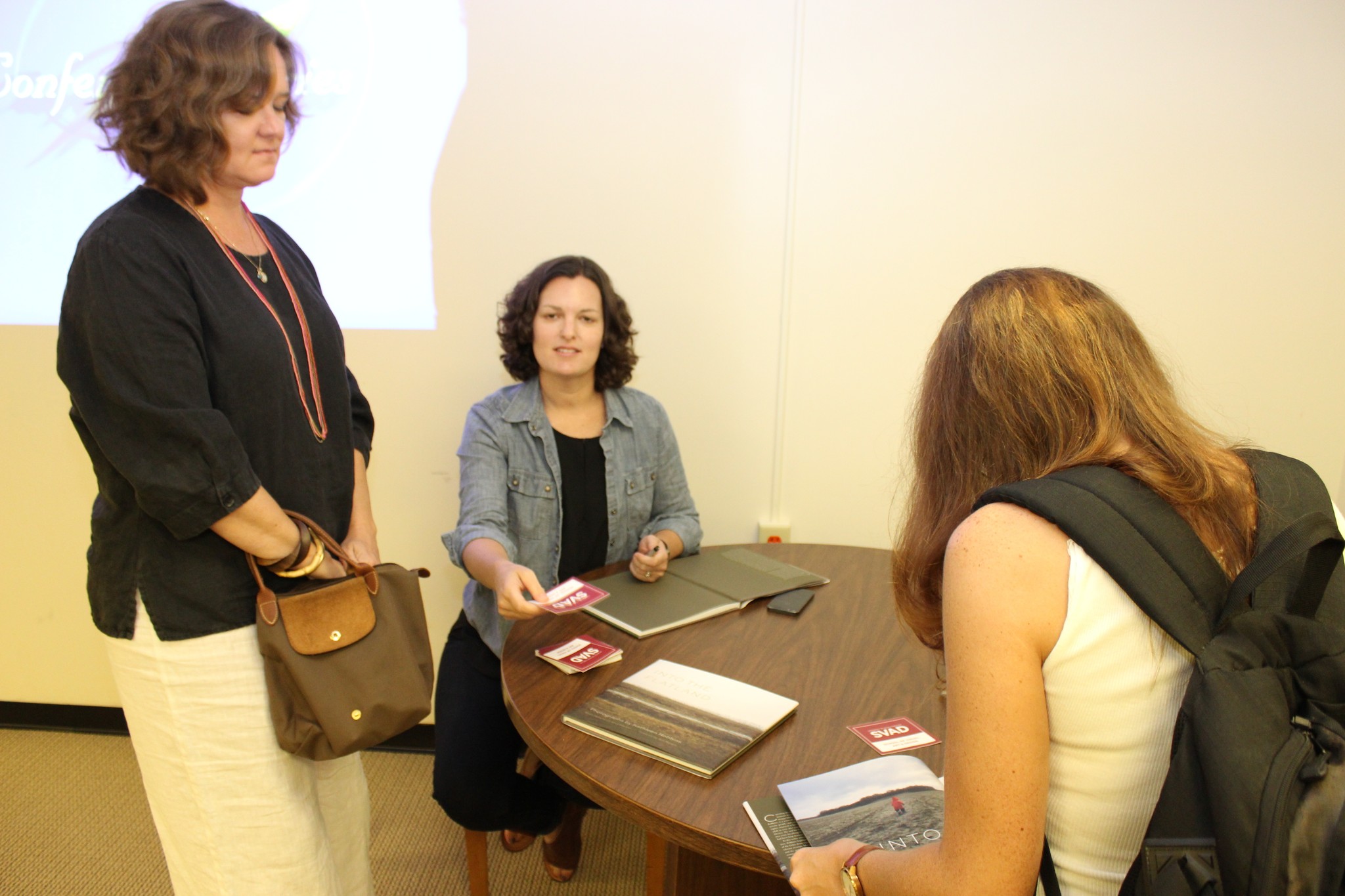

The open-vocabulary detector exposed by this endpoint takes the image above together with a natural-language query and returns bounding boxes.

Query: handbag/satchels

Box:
[248,509,435,761]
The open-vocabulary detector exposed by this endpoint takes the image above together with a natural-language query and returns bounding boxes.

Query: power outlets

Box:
[758,521,791,544]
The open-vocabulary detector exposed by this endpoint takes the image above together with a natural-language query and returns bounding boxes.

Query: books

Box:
[742,755,944,896]
[560,658,800,779]
[578,548,829,640]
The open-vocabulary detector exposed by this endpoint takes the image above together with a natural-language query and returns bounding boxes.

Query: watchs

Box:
[840,844,884,896]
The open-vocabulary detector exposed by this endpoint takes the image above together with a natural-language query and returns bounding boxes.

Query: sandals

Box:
[500,829,536,851]
[542,807,585,882]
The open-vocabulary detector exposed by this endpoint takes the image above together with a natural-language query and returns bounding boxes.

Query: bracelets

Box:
[271,526,324,578]
[660,539,670,556]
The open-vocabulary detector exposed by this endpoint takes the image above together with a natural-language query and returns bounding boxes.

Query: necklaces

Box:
[186,195,268,282]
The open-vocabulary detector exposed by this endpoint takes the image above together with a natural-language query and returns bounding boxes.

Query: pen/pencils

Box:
[645,546,660,557]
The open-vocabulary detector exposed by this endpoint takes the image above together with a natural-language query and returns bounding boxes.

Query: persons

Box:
[55,0,381,896]
[432,256,702,879]
[789,267,1345,896]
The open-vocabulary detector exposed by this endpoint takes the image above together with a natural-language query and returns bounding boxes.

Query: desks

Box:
[503,544,947,896]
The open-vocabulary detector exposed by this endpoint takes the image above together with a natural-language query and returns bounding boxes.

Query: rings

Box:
[645,571,651,577]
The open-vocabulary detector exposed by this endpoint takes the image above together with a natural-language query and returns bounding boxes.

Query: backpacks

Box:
[971,449,1345,896]
